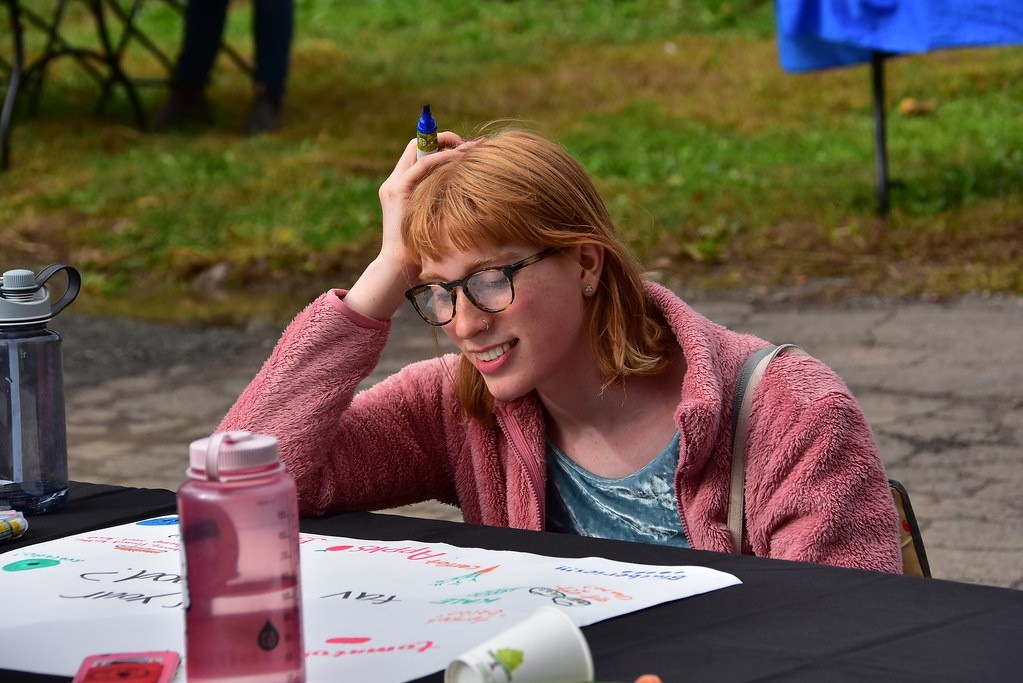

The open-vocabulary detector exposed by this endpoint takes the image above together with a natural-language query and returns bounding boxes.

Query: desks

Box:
[0,479,1023,683]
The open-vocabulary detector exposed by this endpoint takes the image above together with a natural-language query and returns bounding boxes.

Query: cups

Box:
[445,607,597,683]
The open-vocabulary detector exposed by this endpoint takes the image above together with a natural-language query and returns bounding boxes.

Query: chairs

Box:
[887,479,932,578]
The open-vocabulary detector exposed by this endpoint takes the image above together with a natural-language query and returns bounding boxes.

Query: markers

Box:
[416,105,440,160]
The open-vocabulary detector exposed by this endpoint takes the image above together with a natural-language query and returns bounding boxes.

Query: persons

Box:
[168,0,293,133]
[209,119,902,575]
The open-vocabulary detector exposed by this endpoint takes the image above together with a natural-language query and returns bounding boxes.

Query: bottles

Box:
[0,263,82,515]
[178,431,306,683]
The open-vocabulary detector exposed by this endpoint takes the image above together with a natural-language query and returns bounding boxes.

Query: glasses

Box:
[404,245,570,326]
